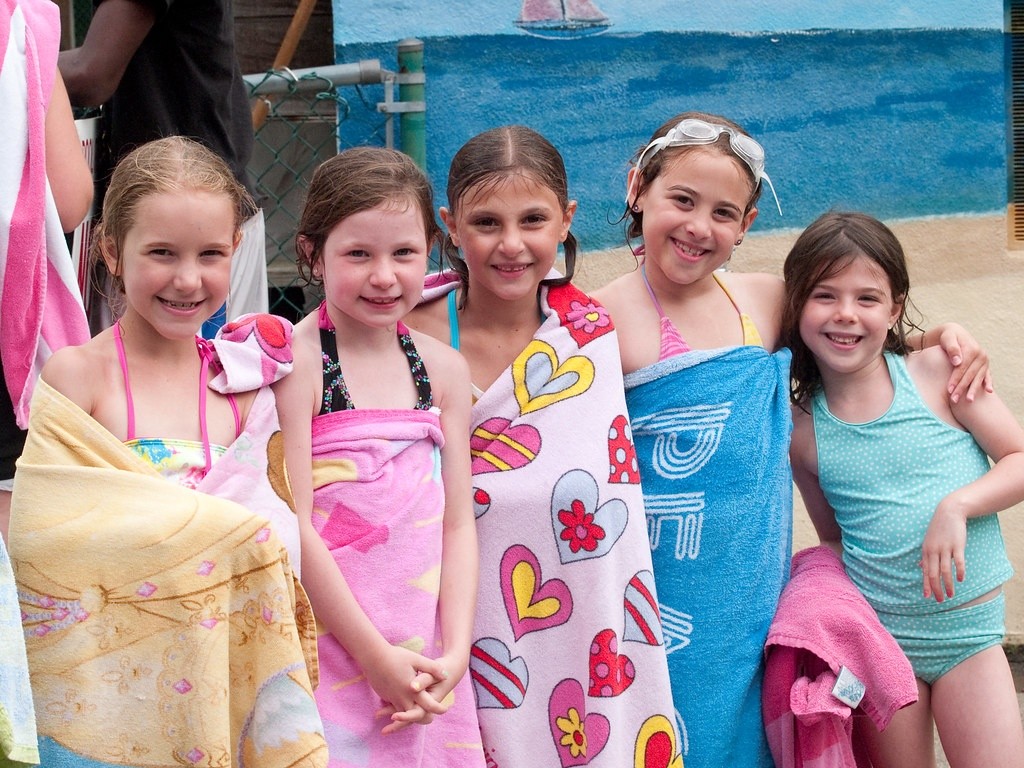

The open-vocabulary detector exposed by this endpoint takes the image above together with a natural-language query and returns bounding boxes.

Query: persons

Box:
[273,144,487,768]
[59,0,270,323]
[786,214,1024,768]
[585,113,992,768]
[395,127,684,768]
[0,1,94,537]
[5,136,328,768]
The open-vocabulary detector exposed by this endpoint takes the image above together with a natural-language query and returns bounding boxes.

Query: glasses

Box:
[662,118,764,171]
